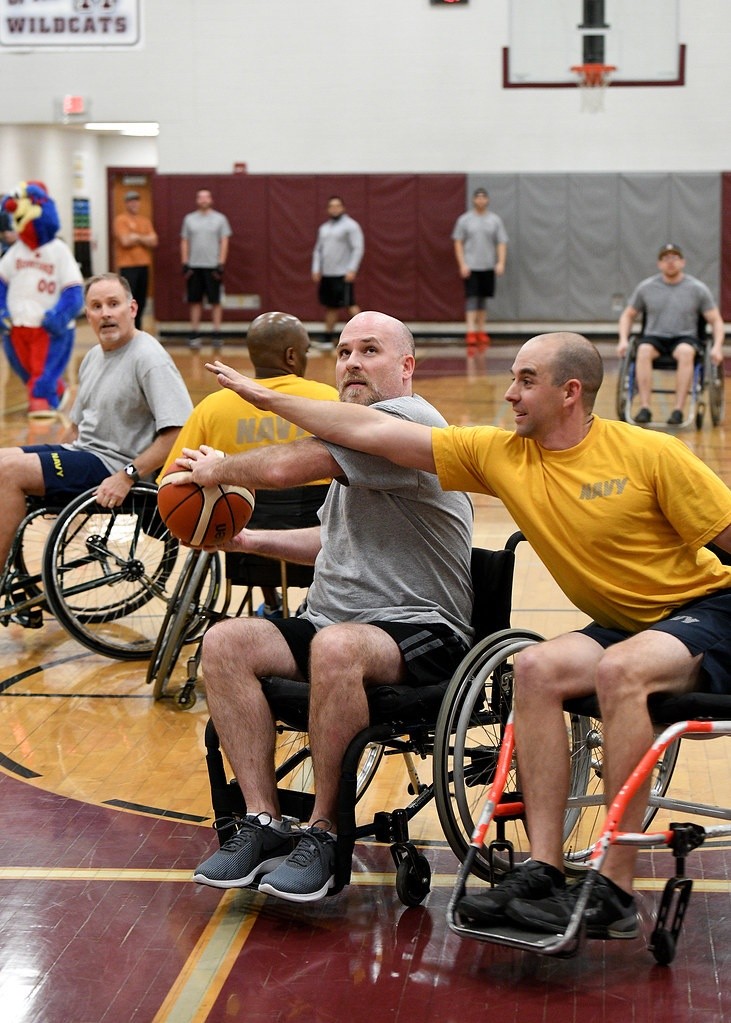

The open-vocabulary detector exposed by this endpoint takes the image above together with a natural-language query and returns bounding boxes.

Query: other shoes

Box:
[633,409,652,424]
[667,410,683,424]
[207,330,221,343]
[478,332,490,344]
[253,592,290,622]
[310,333,334,349]
[465,332,477,345]
[188,332,198,343]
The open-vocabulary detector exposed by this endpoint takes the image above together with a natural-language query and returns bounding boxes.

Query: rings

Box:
[110,502,115,505]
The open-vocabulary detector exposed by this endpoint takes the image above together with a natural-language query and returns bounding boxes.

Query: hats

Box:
[658,243,682,262]
[124,190,141,202]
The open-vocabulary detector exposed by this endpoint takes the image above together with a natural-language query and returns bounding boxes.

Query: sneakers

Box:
[507,871,640,939]
[459,859,567,923]
[192,813,303,889]
[258,818,337,903]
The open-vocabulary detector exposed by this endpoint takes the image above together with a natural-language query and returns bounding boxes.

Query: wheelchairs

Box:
[445,543,730,967]
[204,530,592,908]
[0,476,222,662]
[147,484,330,711]
[615,308,725,431]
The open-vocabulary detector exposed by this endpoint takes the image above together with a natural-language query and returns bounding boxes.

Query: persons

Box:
[450,188,508,344]
[620,243,724,424]
[179,189,233,343]
[156,313,343,618]
[0,273,187,574]
[310,196,364,347]
[171,311,473,900]
[201,329,731,940]
[113,191,158,330]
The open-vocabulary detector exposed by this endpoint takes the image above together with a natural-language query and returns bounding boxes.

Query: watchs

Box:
[124,463,141,481]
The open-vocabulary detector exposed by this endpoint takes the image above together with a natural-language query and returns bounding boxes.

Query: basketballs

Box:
[156,449,256,547]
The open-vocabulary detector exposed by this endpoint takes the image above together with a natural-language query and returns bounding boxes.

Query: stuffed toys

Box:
[0,179,84,417]
